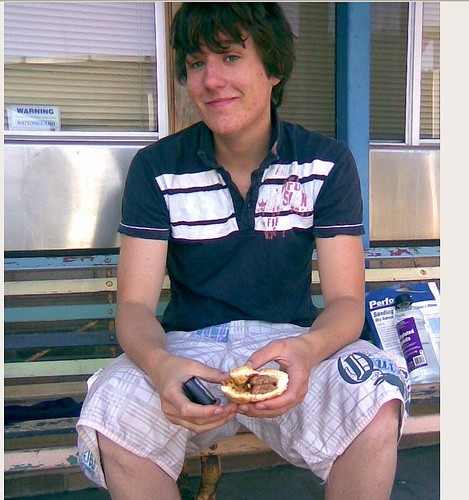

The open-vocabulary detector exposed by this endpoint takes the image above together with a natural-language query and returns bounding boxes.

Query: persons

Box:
[74,2,411,500]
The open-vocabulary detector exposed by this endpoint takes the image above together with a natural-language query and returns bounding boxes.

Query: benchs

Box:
[5,246,439,499]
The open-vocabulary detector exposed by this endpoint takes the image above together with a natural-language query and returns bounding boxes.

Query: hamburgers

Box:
[220,366,289,404]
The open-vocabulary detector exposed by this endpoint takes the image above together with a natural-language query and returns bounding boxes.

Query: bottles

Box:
[393,294,440,385]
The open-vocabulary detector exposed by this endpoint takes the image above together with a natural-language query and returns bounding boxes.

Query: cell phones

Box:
[184,376,216,405]
